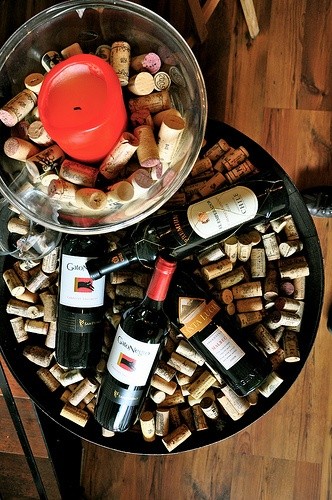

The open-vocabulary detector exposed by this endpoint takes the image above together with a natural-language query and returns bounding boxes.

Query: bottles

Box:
[51,228,107,371]
[84,178,293,281]
[95,255,175,433]
[153,259,275,402]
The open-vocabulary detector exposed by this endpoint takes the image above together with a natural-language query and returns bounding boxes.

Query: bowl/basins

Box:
[0,0,208,241]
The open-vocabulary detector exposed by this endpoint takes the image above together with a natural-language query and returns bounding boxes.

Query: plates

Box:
[2,115,321,458]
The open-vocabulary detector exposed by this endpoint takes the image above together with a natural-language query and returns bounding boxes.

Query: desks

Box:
[1,115,324,457]
